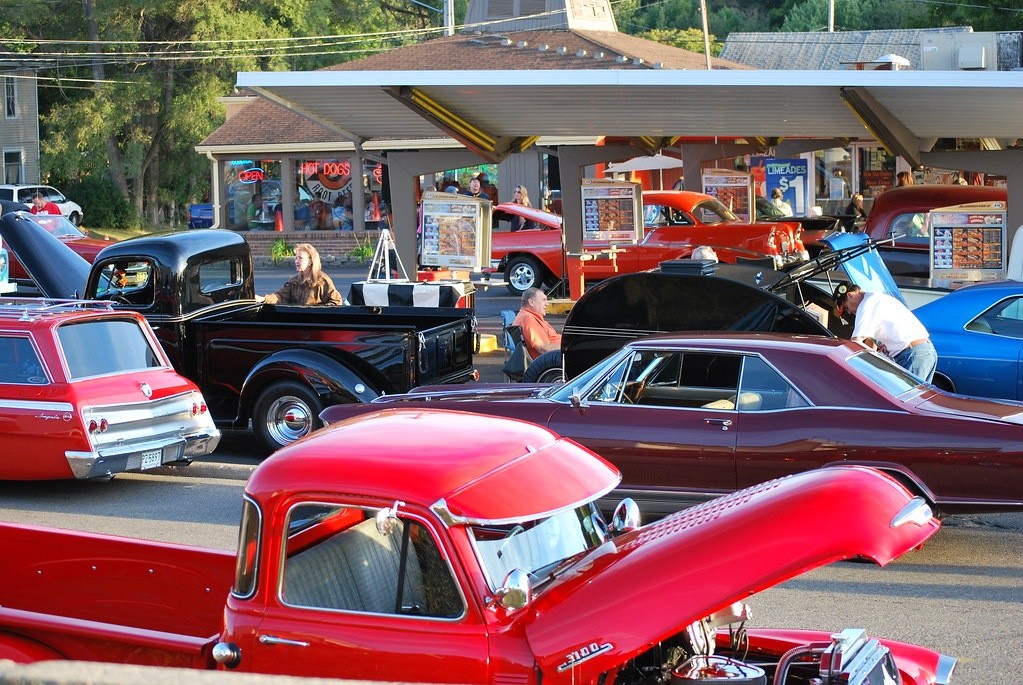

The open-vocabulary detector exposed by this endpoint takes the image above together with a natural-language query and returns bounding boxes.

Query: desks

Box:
[346,280,475,308]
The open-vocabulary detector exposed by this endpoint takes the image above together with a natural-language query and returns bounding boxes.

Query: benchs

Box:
[286,518,432,616]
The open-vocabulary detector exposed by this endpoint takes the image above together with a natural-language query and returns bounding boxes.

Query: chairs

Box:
[502,307,534,384]
[730,391,763,409]
[702,397,737,409]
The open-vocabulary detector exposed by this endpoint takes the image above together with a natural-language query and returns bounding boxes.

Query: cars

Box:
[0,216,121,286]
[319,331,1023,563]
[0,183,83,227]
[754,193,845,233]
[0,296,221,480]
[481,190,810,297]
[865,184,1008,287]
[816,231,1023,400]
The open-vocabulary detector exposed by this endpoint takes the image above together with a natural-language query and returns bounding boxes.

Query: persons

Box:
[462,175,493,201]
[246,190,390,231]
[761,188,794,218]
[263,243,343,306]
[832,280,938,384]
[823,168,852,201]
[894,171,930,237]
[445,186,459,194]
[416,185,439,271]
[511,287,562,361]
[29,192,63,236]
[843,193,868,234]
[510,184,538,233]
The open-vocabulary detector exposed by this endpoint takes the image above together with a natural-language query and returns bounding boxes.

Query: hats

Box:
[832,281,854,317]
[691,246,718,264]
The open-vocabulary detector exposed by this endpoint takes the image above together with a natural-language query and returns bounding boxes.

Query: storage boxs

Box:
[417,268,452,281]
[476,332,498,353]
[451,271,470,279]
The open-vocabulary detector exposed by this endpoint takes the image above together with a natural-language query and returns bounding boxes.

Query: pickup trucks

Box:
[0,406,958,685]
[0,212,482,454]
[187,179,313,229]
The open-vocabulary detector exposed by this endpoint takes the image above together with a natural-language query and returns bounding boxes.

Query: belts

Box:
[905,338,927,349]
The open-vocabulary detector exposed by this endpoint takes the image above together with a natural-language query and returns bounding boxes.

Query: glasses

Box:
[843,302,848,311]
[514,190,521,192]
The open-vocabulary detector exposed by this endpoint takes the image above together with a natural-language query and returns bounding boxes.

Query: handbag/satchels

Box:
[502,340,534,380]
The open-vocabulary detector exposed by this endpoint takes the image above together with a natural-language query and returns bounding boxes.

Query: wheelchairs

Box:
[500,309,562,382]
